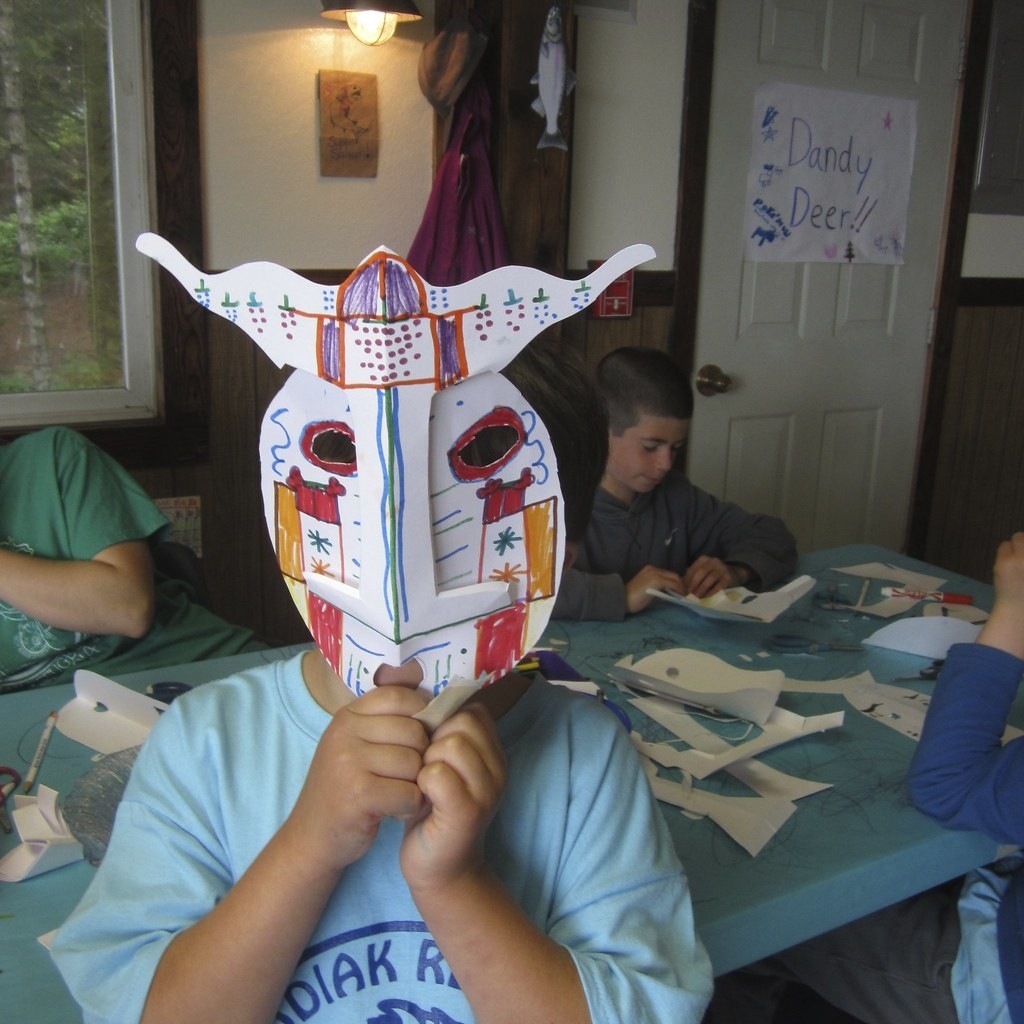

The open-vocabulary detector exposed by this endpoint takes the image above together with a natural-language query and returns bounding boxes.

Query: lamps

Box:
[320,0,423,46]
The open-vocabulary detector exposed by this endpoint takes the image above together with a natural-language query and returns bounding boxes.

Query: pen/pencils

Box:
[854,577,870,612]
[881,585,975,607]
[22,710,61,796]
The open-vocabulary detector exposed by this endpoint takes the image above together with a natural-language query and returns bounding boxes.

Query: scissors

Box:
[0,766,20,834]
[768,632,866,657]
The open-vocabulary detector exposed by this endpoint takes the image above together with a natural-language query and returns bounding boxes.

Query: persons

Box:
[0,426,277,694]
[713,526,1024,1024]
[46,336,715,1024]
[548,344,801,621]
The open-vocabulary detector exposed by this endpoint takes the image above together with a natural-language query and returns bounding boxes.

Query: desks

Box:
[0,545,1024,1024]
